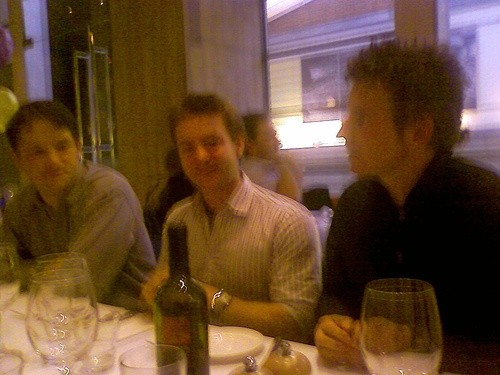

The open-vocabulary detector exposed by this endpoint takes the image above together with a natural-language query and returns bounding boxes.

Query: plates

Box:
[207,325,265,363]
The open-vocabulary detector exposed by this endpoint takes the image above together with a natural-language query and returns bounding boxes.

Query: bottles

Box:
[151,219,211,375]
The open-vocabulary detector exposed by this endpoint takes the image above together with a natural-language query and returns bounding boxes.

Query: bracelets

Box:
[208,288,232,324]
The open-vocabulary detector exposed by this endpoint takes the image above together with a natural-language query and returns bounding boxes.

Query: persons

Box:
[313,34,500,375]
[141,93,324,344]
[239,113,303,205]
[0,100,158,312]
[0,26,27,207]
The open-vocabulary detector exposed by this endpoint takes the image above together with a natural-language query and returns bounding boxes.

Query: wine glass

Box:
[0,242,24,375]
[25,267,99,375]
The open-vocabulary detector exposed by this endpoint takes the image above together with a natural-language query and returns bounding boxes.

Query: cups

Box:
[359,278,442,375]
[82,308,121,371]
[34,250,93,364]
[117,345,187,375]
